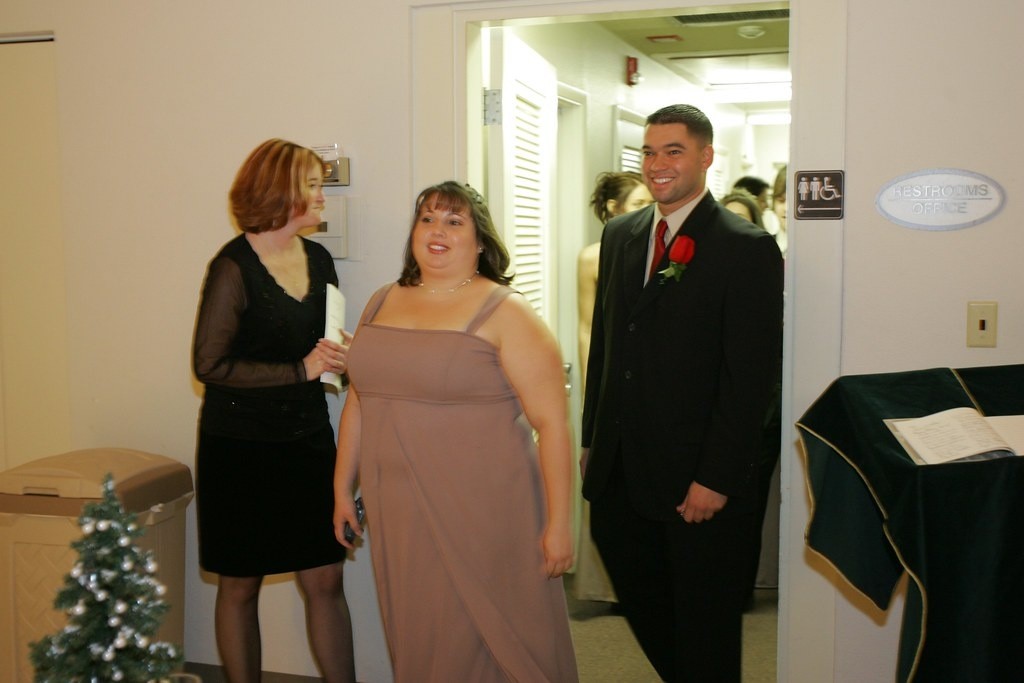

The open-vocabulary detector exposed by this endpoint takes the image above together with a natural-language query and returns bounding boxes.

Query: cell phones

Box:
[343,498,366,543]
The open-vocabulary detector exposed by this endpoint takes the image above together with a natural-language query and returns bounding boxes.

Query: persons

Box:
[193,137,354,683]
[578,103,788,683]
[334,180,578,683]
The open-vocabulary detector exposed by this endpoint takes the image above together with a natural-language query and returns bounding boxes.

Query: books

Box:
[883,407,1023,466]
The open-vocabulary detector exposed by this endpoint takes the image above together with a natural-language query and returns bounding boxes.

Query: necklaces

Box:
[418,269,480,295]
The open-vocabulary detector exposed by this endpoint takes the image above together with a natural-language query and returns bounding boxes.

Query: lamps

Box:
[736,26,766,39]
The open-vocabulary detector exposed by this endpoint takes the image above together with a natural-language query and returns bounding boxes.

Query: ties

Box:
[649,220,668,280]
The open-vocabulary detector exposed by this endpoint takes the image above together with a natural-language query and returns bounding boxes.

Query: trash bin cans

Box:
[0,448,196,683]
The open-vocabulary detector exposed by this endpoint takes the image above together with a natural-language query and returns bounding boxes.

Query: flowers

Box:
[657,235,695,285]
